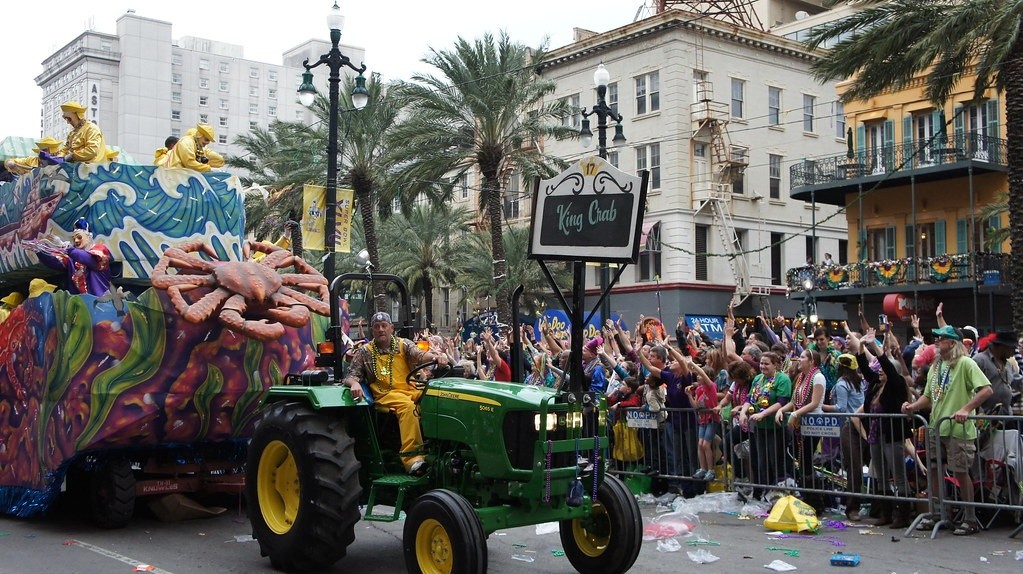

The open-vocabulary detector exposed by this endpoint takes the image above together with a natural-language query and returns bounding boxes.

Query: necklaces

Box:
[372,335,396,392]
[994,357,1011,389]
[593,435,600,502]
[932,357,952,401]
[791,367,821,409]
[732,384,749,432]
[546,441,552,502]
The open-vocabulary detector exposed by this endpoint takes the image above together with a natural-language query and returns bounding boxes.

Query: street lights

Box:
[795,277,819,337]
[296,1,371,291]
[578,61,629,339]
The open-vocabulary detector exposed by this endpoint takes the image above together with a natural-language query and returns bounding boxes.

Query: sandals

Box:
[953,519,980,536]
[915,515,945,531]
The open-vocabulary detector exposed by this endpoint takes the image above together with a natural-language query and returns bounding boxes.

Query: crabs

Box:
[152,239,330,340]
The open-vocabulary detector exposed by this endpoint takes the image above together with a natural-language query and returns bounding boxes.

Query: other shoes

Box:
[875,511,894,526]
[889,516,911,530]
[848,509,861,521]
[408,461,427,475]
[702,470,715,481]
[692,468,707,478]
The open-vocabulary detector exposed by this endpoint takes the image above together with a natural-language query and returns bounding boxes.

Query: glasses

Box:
[61,115,79,122]
[934,335,950,342]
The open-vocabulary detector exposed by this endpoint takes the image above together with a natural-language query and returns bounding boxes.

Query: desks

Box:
[839,163,867,180]
[931,148,962,165]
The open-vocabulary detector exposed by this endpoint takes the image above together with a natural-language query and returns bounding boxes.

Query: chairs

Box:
[943,427,1019,528]
[813,161,837,183]
[919,149,931,168]
[861,156,877,178]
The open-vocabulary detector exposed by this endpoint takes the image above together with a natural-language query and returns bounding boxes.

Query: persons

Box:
[154,124,224,172]
[342,311,448,475]
[804,253,813,265]
[412,328,460,378]
[23,217,114,297]
[821,253,833,266]
[465,325,511,382]
[520,316,606,415]
[597,314,720,481]
[49,101,107,164]
[717,304,1023,536]
[357,318,369,345]
[3,137,62,178]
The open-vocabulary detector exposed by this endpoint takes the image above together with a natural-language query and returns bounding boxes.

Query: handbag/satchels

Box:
[612,418,644,462]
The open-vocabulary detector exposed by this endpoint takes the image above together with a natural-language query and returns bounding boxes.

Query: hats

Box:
[31,146,40,153]
[830,336,846,348]
[371,311,392,328]
[72,216,90,231]
[34,136,62,153]
[60,101,87,119]
[835,354,859,370]
[989,330,1019,349]
[29,278,57,297]
[196,123,216,142]
[964,326,979,338]
[0,292,24,307]
[932,326,960,341]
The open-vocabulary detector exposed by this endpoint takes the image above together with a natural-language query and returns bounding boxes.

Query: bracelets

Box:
[545,334,549,339]
[668,347,672,352]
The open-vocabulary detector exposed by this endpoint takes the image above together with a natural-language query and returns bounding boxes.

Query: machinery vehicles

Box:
[243,247,644,574]
